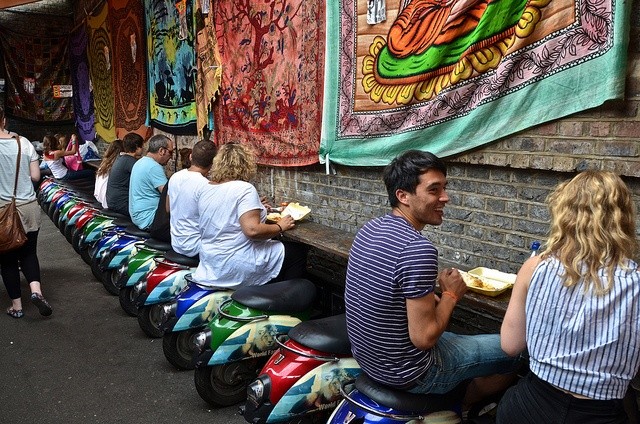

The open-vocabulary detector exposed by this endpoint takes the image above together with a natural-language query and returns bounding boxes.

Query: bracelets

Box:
[276,223,283,234]
[72,141,76,144]
[441,291,459,301]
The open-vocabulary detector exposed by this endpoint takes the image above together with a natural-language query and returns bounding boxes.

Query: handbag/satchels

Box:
[0,136,29,253]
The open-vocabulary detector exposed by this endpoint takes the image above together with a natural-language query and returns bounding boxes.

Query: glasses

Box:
[158,145,175,156]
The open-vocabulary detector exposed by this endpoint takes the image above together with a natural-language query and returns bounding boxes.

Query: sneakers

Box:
[30,292,54,316]
[5,306,24,318]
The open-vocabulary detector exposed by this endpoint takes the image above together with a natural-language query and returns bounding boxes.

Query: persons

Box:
[1,105,54,319]
[106,132,145,217]
[93,139,125,210]
[165,139,217,259]
[42,133,96,182]
[496,169,639,423]
[191,141,309,291]
[344,148,525,423]
[128,133,173,232]
